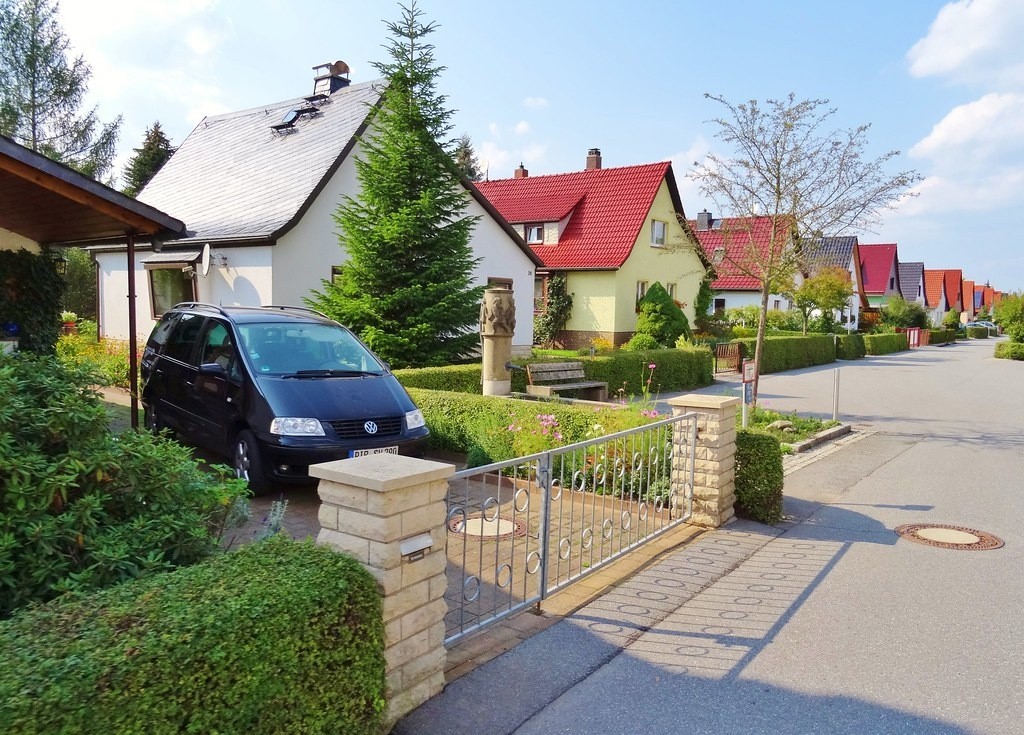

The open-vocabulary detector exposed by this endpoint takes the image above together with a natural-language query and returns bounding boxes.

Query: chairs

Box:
[283,338,317,371]
[175,326,212,363]
[208,334,246,372]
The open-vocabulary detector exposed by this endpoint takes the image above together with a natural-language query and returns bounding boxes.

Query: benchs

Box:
[526,362,609,402]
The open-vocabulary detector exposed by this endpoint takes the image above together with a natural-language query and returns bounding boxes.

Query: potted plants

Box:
[59,310,77,327]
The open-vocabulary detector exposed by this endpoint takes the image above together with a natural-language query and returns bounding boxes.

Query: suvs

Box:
[137,300,431,498]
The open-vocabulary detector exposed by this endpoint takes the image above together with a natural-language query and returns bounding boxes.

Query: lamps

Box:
[50,251,66,275]
